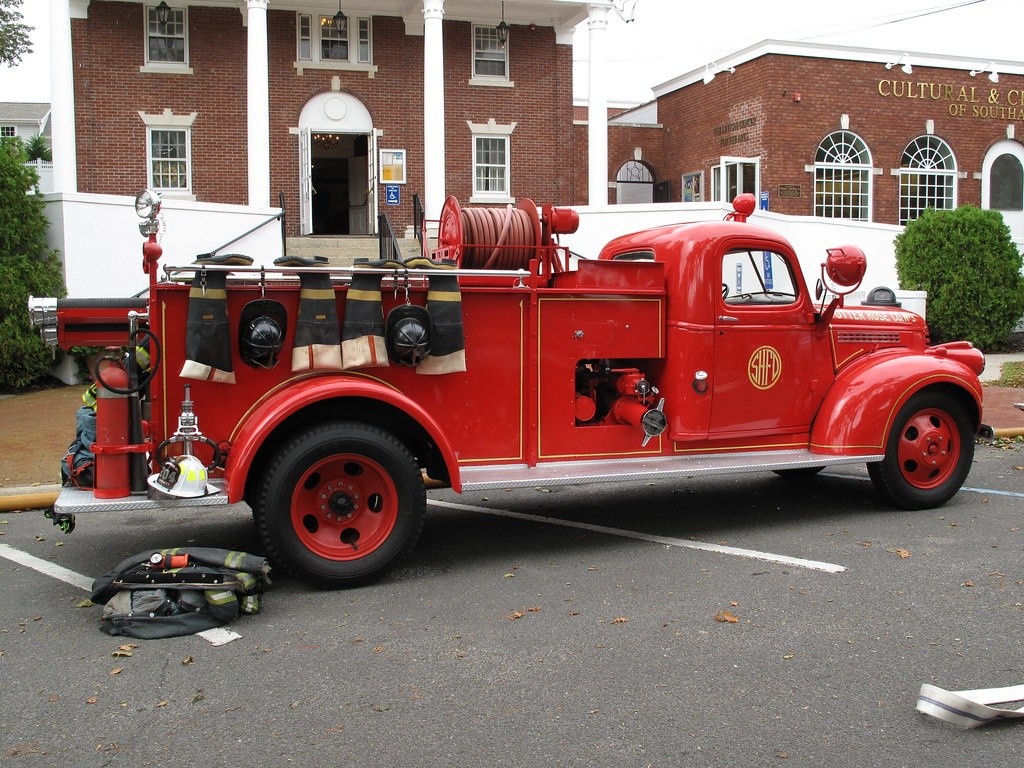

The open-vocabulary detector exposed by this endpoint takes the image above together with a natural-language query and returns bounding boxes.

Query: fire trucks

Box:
[24,175,987,588]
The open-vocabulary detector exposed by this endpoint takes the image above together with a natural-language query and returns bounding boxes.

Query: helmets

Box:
[385,304,433,366]
[147,455,222,498]
[238,299,288,370]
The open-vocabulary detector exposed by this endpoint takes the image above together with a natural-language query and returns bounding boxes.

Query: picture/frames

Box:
[379,149,406,185]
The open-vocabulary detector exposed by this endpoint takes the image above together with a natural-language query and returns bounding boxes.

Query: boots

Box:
[179,253,255,384]
[341,258,409,371]
[402,257,467,374]
[273,255,343,371]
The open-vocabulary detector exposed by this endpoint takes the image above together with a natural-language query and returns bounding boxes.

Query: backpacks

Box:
[90,548,272,640]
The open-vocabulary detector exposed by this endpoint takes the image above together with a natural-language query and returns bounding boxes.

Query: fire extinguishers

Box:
[94,327,162,499]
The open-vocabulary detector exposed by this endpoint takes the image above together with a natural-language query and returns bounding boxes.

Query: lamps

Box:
[155,0,171,25]
[885,52,912,74]
[313,134,341,150]
[332,0,348,36]
[495,1,510,46]
[703,61,736,85]
[970,62,999,84]
